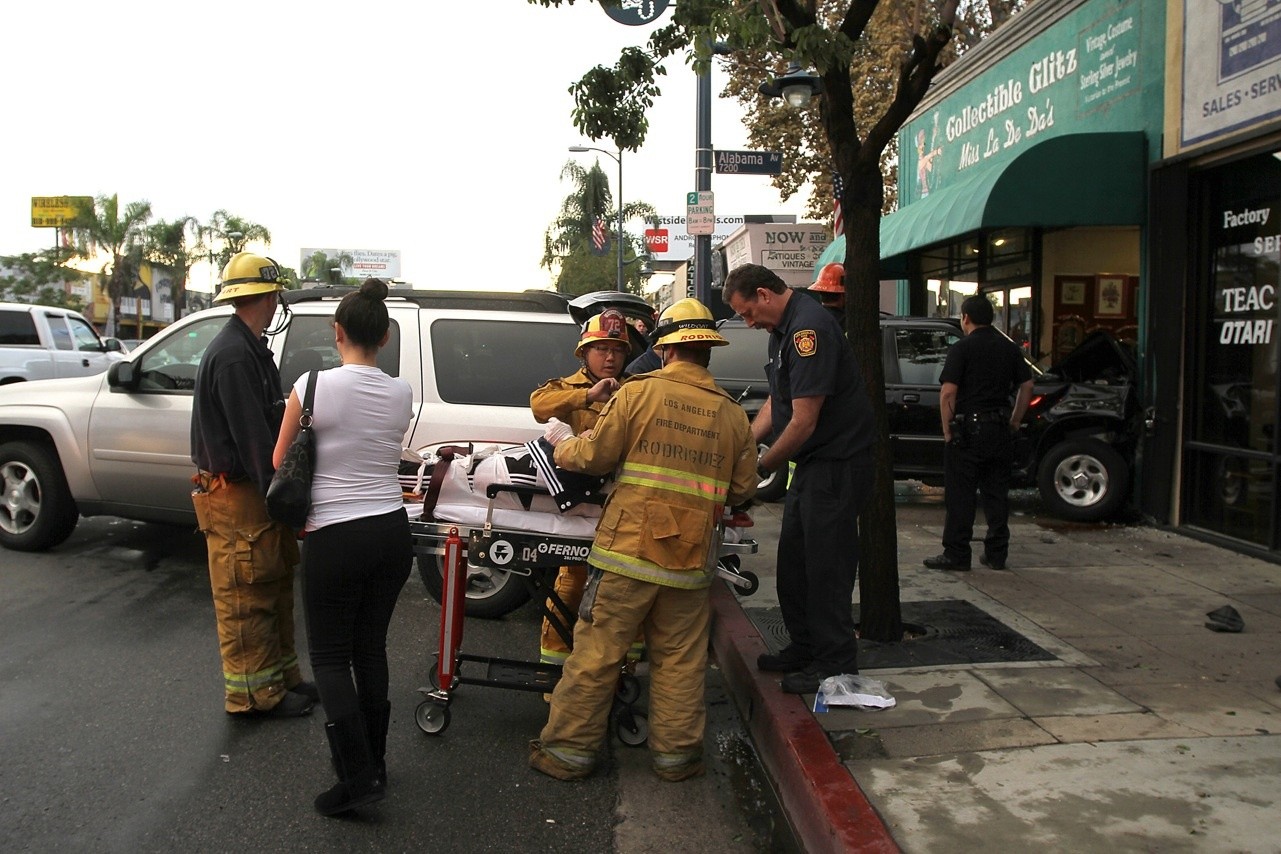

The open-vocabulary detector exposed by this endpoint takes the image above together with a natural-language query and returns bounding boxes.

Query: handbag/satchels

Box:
[266,369,318,524]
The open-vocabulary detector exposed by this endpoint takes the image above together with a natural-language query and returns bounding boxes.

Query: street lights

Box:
[691,28,826,307]
[569,143,623,292]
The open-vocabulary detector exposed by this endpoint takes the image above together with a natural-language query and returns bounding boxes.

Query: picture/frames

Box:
[1093,274,1127,319]
[1061,281,1087,305]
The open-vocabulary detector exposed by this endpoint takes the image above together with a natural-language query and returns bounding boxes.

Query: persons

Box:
[529,298,759,784]
[530,309,645,704]
[164,338,201,366]
[722,262,879,692]
[190,252,321,718]
[625,340,663,374]
[634,319,649,339]
[272,279,415,814]
[924,295,1034,570]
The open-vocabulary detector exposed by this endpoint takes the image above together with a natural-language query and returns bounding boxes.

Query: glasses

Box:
[586,343,627,358]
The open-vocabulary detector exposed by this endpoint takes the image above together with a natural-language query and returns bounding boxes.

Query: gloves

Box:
[542,418,575,448]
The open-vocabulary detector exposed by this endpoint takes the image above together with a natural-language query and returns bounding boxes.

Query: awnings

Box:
[813,130,1148,281]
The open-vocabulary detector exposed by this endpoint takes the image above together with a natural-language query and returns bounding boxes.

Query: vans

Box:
[0,285,658,617]
[0,302,130,386]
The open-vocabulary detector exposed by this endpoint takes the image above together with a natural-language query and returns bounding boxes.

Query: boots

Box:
[314,712,373,816]
[360,695,391,790]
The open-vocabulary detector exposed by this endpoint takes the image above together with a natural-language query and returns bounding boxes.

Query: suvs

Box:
[705,312,1136,524]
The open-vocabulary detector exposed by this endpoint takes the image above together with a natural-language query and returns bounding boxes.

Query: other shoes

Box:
[783,675,824,694]
[980,552,1004,570]
[232,690,313,717]
[924,555,970,571]
[536,668,553,704]
[757,645,808,671]
[530,750,585,780]
[292,683,321,702]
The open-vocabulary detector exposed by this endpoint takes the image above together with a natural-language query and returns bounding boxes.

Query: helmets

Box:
[651,296,732,351]
[574,307,632,359]
[807,262,845,293]
[211,251,286,303]
